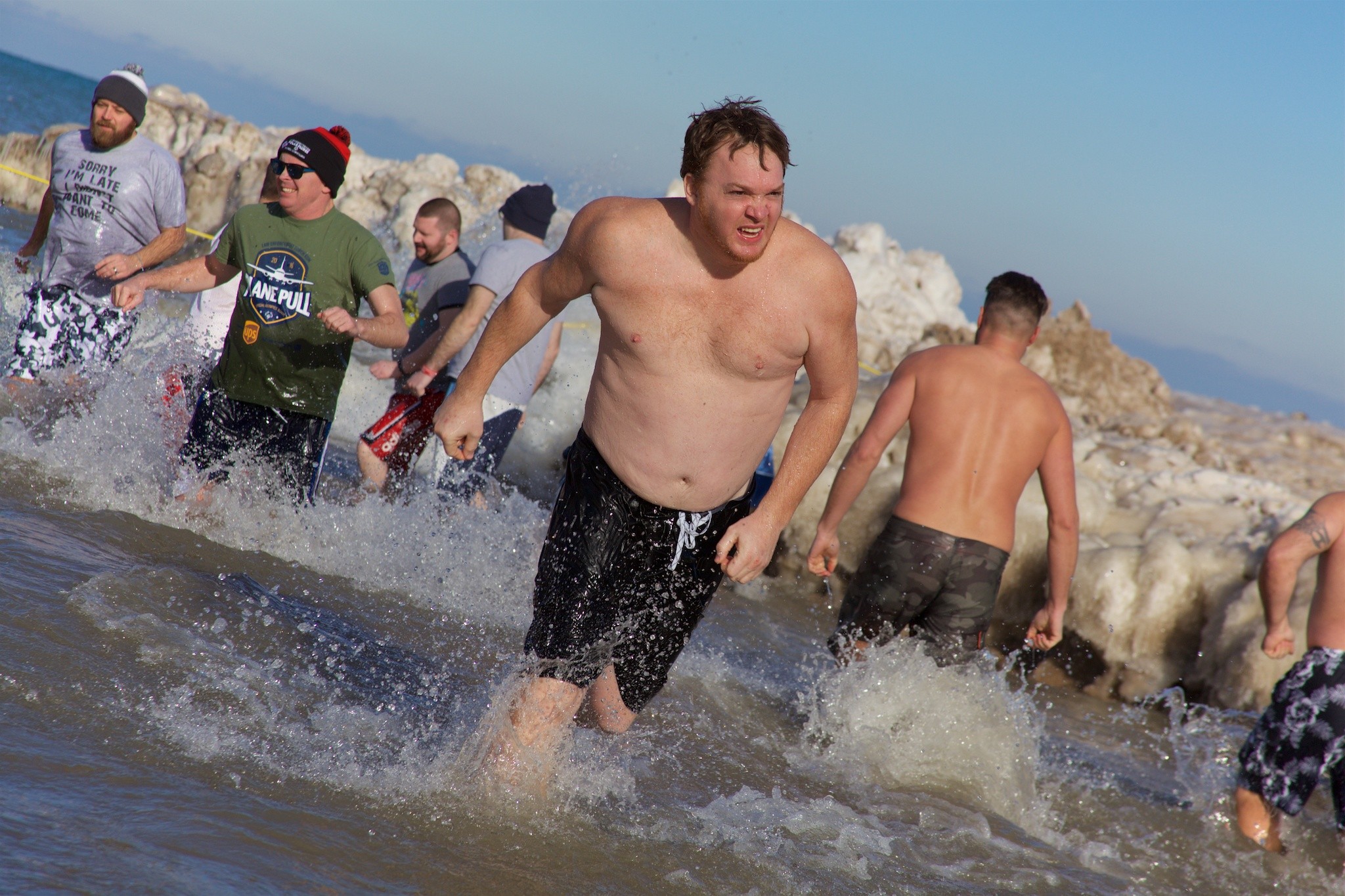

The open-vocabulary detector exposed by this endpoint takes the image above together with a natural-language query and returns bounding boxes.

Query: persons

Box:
[357,197,476,494]
[807,271,1081,666]
[5,63,188,398]
[179,157,280,411]
[111,126,410,514]
[433,98,859,786]
[406,183,567,513]
[1237,491,1345,855]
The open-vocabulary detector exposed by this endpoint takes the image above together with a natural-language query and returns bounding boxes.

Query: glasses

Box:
[270,158,314,181]
[498,209,508,221]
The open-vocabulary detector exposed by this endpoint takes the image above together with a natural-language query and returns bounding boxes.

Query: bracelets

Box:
[135,253,144,271]
[421,364,437,377]
[398,359,406,375]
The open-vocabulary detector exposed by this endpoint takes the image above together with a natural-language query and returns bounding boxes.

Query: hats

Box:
[499,184,557,240]
[277,124,351,198]
[93,64,148,129]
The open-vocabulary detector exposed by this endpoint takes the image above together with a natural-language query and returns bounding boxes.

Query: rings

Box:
[113,267,117,273]
